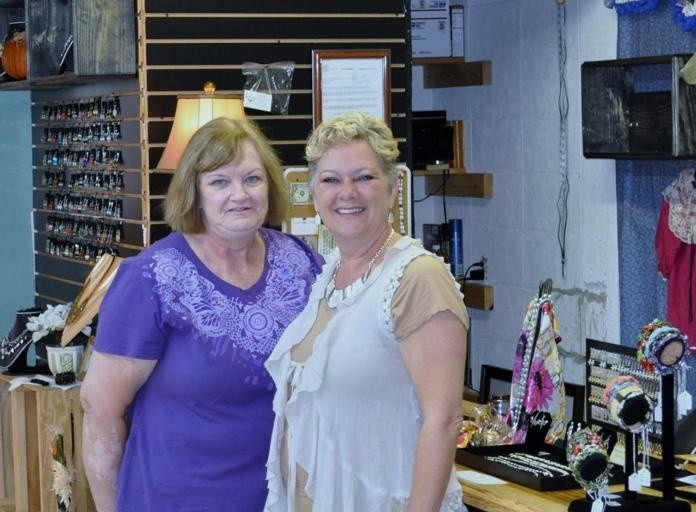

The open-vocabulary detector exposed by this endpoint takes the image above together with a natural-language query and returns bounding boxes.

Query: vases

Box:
[45,343,84,379]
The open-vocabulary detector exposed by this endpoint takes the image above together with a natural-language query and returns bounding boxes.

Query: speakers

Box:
[412,110,447,170]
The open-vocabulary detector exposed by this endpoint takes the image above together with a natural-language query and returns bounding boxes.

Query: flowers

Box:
[22,300,92,347]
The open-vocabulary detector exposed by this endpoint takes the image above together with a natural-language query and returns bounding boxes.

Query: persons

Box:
[263,111,473,512]
[80,117,329,512]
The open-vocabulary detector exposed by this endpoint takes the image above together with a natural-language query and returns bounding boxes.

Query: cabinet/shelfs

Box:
[411,53,494,310]
[0,0,136,92]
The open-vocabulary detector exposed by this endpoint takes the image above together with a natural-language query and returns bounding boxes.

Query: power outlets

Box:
[477,254,491,280]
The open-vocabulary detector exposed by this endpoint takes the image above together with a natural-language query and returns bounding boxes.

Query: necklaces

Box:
[322,228,398,310]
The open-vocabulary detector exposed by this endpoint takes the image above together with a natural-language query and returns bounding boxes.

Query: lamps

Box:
[156,80,247,174]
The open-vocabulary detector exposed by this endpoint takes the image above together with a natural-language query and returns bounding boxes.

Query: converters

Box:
[469,270,484,280]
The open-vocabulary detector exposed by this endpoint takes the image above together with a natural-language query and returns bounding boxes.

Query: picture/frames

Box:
[309,48,394,136]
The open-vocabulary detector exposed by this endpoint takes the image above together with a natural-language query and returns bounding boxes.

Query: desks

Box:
[454,383,695,511]
